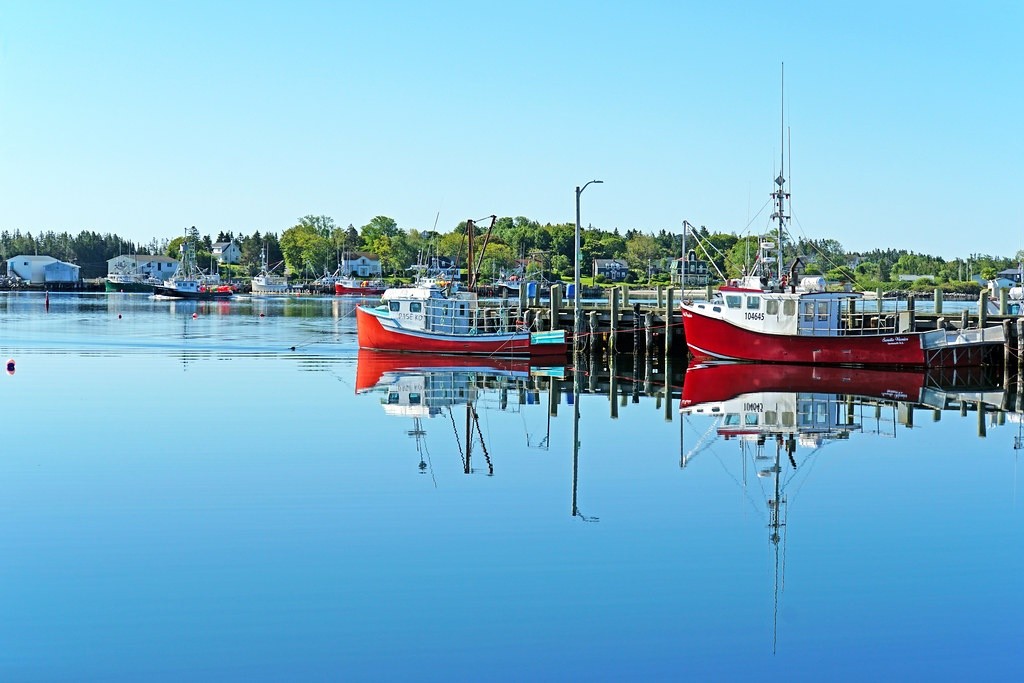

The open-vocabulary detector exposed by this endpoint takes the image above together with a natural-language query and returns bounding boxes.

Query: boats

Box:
[104,242,164,293]
[355,212,568,356]
[679,358,1008,655]
[680,62,1008,369]
[354,347,569,489]
[153,227,233,299]
[292,241,390,294]
[410,237,551,297]
[251,243,288,292]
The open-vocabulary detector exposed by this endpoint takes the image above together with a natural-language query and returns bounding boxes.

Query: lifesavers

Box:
[522,277,525,281]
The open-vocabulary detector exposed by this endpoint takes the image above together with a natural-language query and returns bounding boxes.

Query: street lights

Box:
[574,181,604,349]
[572,349,599,522]
